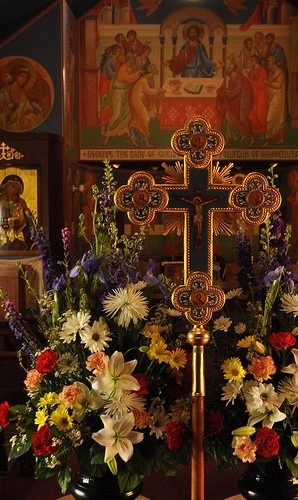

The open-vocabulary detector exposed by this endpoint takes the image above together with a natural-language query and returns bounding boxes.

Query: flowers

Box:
[0,153,297,500]
